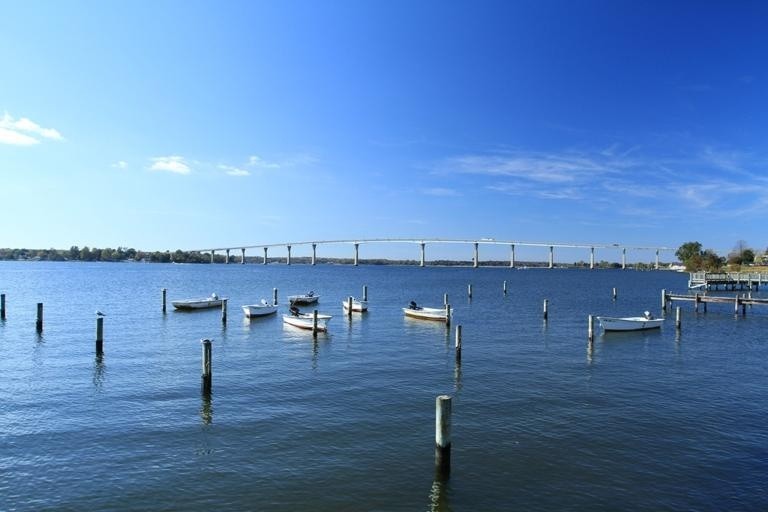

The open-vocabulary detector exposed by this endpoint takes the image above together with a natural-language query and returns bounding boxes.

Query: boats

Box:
[401,301,453,321]
[171,297,229,309]
[342,301,368,312]
[241,304,278,318]
[595,311,664,331]
[282,307,332,333]
[286,291,321,304]
